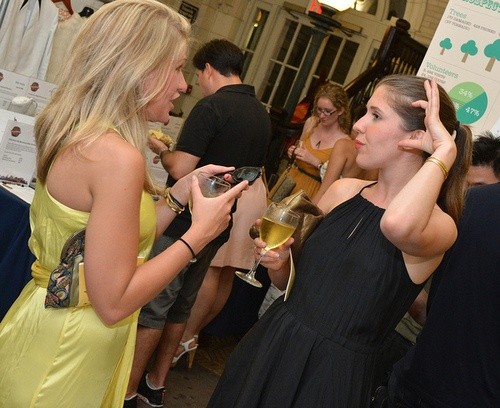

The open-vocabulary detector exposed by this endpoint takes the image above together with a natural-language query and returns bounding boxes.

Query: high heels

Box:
[169,335,198,369]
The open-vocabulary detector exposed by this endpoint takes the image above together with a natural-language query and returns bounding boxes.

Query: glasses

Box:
[310,108,339,116]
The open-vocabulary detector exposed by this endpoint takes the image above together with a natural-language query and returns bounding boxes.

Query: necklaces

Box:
[316,126,339,149]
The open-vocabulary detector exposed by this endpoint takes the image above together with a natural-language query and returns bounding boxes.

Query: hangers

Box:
[53,0,94,19]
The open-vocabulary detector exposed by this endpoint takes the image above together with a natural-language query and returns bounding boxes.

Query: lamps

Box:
[308,0,356,31]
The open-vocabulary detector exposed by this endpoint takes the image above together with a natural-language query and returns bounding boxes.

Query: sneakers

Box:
[124,395,139,408]
[137,372,167,407]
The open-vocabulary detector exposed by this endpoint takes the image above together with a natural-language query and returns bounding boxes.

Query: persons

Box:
[0,0,249,408]
[207,74,473,407]
[124,38,500,408]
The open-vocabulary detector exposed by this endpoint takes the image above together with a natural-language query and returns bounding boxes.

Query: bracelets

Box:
[163,187,185,215]
[159,148,170,158]
[319,161,324,170]
[178,238,197,266]
[422,156,449,180]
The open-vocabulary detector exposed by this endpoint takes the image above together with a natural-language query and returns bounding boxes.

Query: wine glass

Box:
[288,140,304,169]
[189,171,231,262]
[235,202,300,288]
